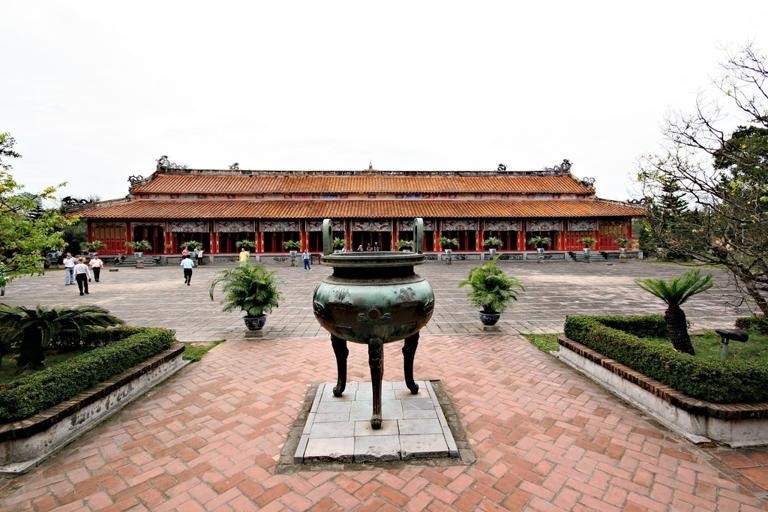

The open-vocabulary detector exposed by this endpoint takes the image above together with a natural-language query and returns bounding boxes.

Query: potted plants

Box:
[81,236,346,258]
[395,236,631,255]
[455,252,527,325]
[208,259,285,330]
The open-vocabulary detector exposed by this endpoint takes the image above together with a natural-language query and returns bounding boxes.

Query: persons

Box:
[55,249,64,265]
[197,248,205,266]
[372,241,380,251]
[62,252,77,286]
[238,247,250,262]
[301,249,312,271]
[72,256,92,296]
[89,254,104,283]
[356,243,363,252]
[367,242,373,251]
[181,245,188,258]
[180,254,195,286]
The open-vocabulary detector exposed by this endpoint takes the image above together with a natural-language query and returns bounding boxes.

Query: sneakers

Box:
[184,279,188,283]
[84,291,89,294]
[70,281,75,285]
[65,283,69,286]
[80,292,84,296]
[187,283,190,285]
